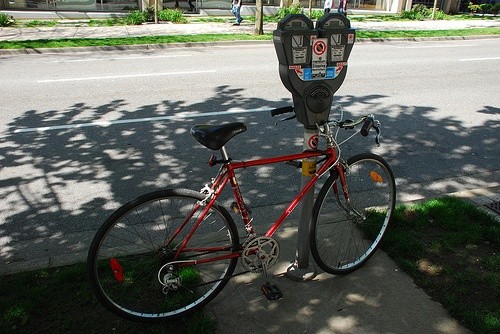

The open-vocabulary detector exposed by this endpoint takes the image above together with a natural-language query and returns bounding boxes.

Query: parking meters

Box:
[275,10,356,282]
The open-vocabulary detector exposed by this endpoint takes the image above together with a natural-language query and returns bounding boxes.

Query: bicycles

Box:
[86,103,398,325]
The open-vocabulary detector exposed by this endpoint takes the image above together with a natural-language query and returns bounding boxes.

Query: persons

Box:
[231,0,243,26]
[324,0,334,15]
[338,0,347,17]
